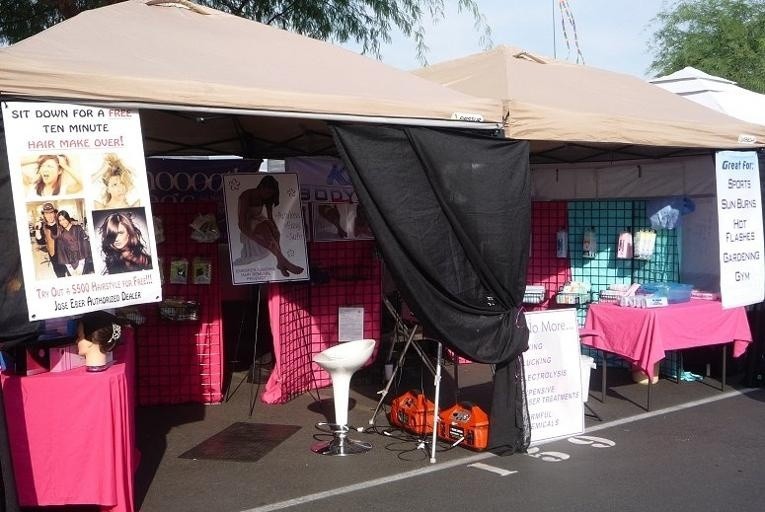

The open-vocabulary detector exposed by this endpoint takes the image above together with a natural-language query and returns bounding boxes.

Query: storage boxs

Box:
[643,282,694,303]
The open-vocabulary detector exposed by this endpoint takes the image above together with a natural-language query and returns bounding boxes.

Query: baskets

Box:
[598,294,622,303]
[555,292,592,305]
[116,307,149,327]
[522,293,547,304]
[157,294,202,322]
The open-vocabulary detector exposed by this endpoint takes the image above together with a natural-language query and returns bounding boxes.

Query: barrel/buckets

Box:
[631,361,660,385]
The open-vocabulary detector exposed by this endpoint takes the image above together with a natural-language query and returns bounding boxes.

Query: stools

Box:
[313,339,375,455]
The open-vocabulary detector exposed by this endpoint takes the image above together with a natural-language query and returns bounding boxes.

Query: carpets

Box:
[176,423,303,466]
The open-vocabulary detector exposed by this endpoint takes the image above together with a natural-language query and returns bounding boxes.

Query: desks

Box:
[590,301,750,411]
[2,327,136,512]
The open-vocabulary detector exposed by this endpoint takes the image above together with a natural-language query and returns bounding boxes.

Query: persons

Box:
[21,153,84,196]
[54,208,92,276]
[92,152,141,208]
[238,176,304,278]
[74,311,124,368]
[100,210,152,274]
[33,202,68,278]
